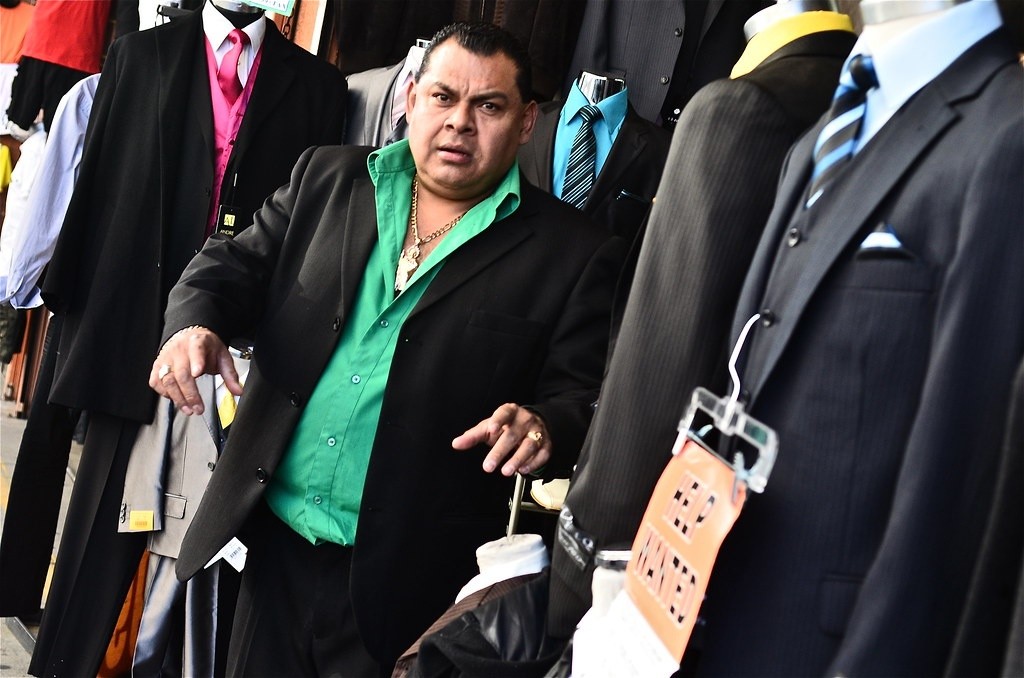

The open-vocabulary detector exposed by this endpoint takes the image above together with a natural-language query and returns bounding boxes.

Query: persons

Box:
[542,0,861,642]
[341,37,437,148]
[518,71,673,239]
[25,0,350,678]
[670,0,1023,678]
[147,17,628,678]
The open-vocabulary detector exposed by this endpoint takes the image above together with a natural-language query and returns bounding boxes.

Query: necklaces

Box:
[394,172,471,293]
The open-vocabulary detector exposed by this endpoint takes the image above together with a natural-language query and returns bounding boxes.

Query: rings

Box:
[158,364,171,380]
[527,430,544,450]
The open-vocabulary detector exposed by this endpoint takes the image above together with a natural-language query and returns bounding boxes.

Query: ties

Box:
[216,28,250,107]
[386,113,409,149]
[560,106,605,213]
[220,385,240,429]
[807,54,875,211]
